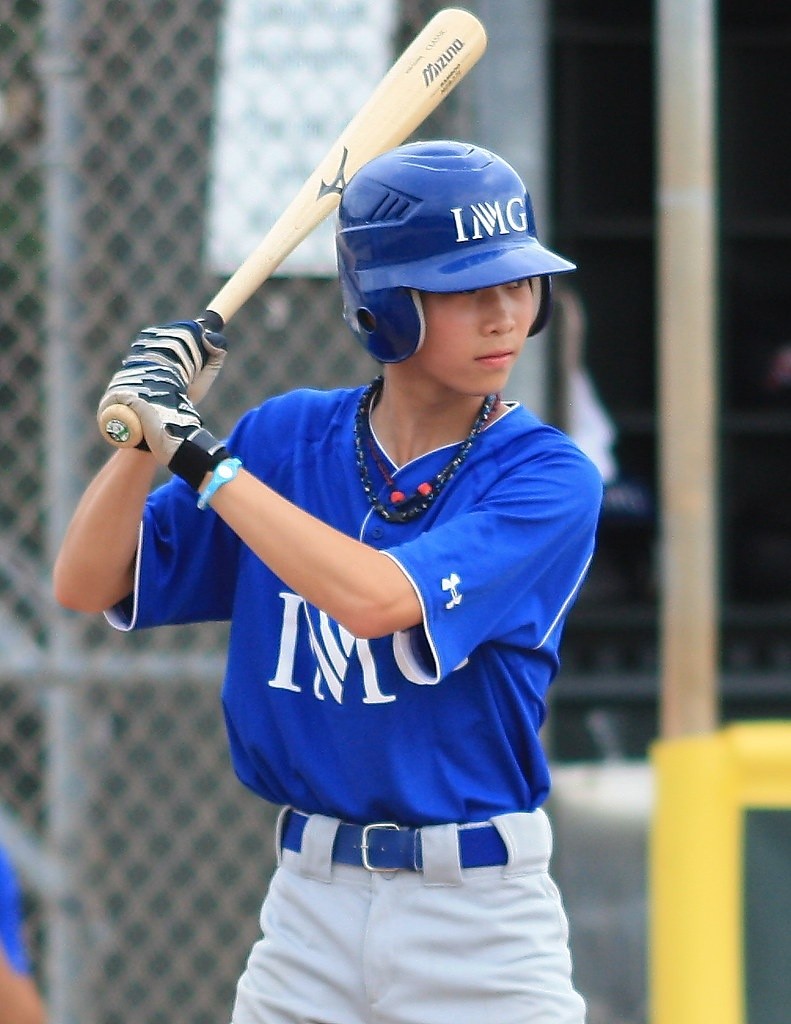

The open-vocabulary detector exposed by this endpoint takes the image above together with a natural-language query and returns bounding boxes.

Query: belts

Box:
[281,810,508,871]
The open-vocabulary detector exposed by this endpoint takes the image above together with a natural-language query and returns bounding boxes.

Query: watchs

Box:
[197,457,242,509]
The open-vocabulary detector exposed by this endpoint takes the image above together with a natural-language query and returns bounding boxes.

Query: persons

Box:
[0,848,49,1024]
[52,141,601,1023]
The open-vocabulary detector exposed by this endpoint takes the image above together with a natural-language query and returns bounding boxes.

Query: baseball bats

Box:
[94,8,488,451]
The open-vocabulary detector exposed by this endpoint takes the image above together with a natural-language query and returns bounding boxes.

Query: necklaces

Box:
[354,376,500,523]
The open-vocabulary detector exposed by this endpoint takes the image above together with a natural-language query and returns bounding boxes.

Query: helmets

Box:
[336,140,579,362]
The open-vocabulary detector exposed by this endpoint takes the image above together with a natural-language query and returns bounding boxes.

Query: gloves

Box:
[125,319,226,408]
[96,362,226,492]
